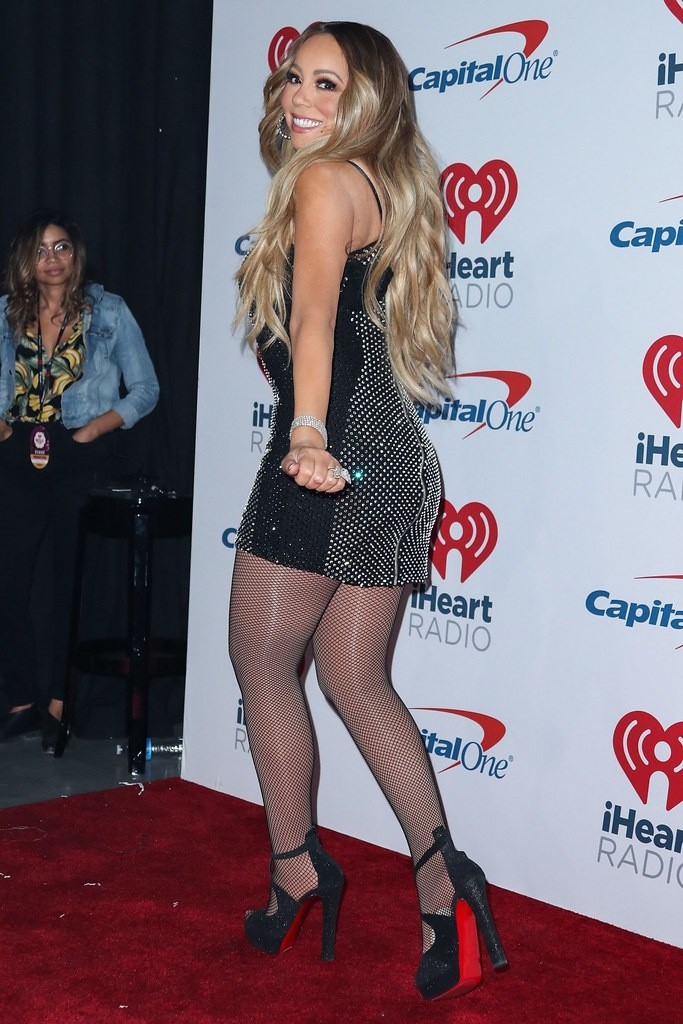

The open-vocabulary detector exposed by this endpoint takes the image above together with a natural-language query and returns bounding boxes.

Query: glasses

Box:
[39,241,75,264]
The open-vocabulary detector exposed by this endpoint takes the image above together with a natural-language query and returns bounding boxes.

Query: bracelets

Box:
[289,416,328,451]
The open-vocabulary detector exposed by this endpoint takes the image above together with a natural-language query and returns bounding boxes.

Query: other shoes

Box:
[43,703,70,754]
[0,703,40,741]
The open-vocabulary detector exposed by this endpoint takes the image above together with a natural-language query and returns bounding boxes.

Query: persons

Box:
[0,207,161,756]
[228,22,509,1001]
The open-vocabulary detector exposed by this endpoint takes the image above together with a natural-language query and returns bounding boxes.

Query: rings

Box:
[328,466,352,484]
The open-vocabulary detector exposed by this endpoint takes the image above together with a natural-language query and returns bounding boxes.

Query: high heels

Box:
[412,829,508,1004]
[243,827,341,964]
